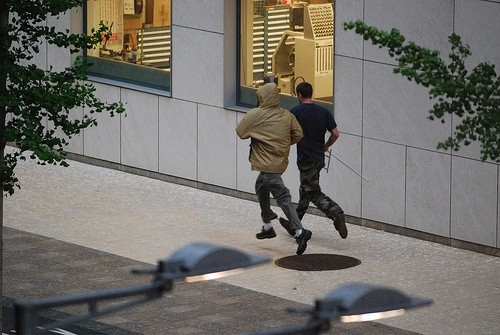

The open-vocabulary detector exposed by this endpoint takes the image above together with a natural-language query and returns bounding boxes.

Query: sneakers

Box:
[256,225,277,241]
[279,217,296,236]
[294,228,312,255]
[334,212,349,240]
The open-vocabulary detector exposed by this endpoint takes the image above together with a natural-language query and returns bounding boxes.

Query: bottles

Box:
[126,51,132,64]
[131,48,137,63]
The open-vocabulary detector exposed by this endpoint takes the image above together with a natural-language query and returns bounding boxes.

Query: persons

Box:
[235,82,313,256]
[278,82,348,238]
